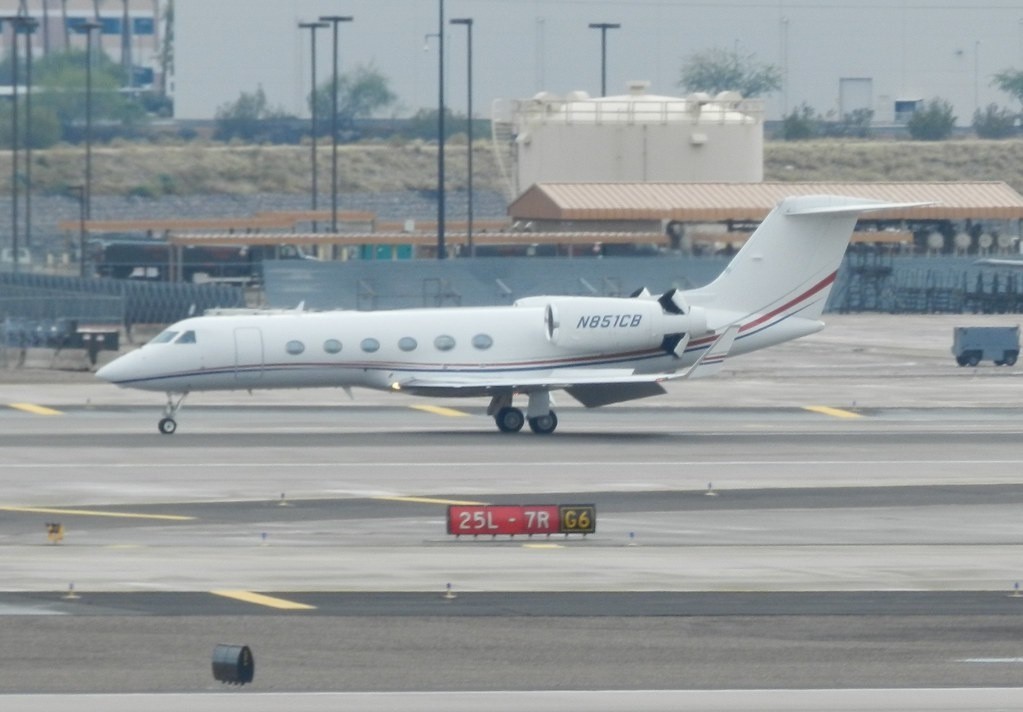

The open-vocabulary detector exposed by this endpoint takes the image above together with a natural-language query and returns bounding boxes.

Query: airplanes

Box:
[95,192,942,434]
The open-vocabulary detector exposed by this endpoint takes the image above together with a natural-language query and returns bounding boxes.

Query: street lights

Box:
[422,31,447,259]
[76,20,102,212]
[588,21,621,99]
[449,18,474,257]
[298,23,327,260]
[319,16,354,233]
[14,17,40,240]
